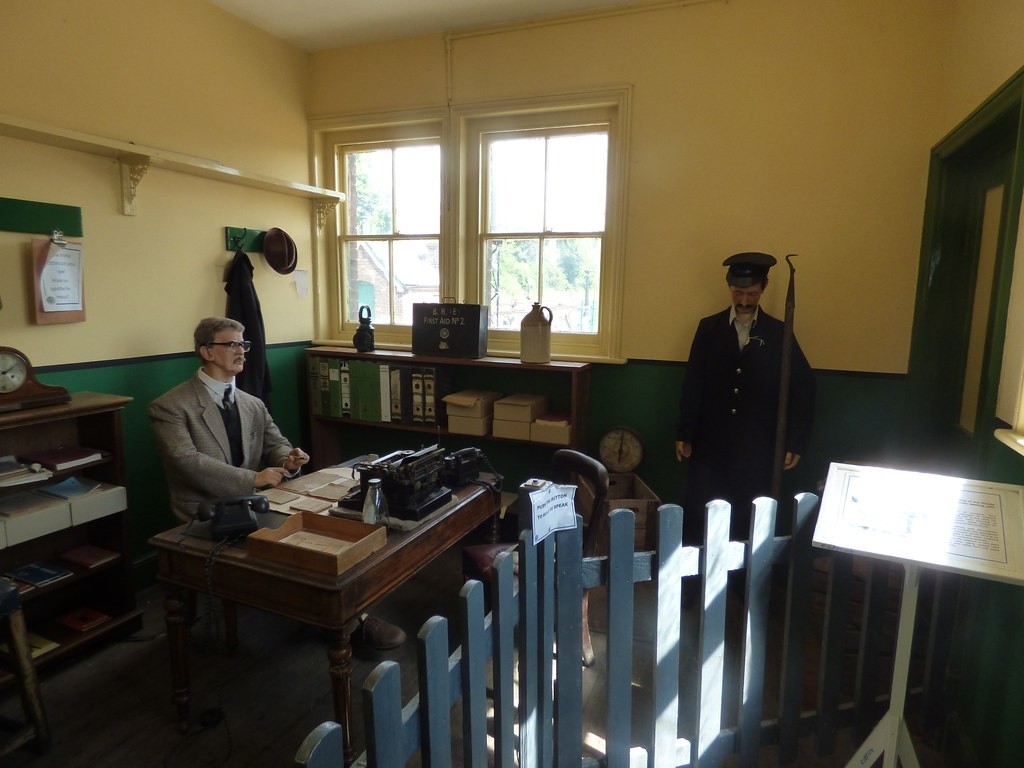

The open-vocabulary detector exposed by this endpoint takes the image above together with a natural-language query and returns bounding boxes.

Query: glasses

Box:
[208,341,252,353]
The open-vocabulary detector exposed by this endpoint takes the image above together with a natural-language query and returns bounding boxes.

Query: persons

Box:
[149,315,409,648]
[676,249,814,636]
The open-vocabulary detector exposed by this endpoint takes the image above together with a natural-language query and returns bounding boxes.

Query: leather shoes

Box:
[355,617,406,650]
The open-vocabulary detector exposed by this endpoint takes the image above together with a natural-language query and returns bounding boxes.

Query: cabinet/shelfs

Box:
[304,345,593,486]
[0,391,150,689]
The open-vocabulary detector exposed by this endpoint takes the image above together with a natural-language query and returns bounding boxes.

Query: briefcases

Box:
[411,297,489,360]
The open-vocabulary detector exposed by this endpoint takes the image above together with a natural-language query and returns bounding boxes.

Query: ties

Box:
[222,387,234,412]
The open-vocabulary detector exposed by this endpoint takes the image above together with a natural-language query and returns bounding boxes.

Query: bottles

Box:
[362,478,390,534]
[520,302,553,363]
[353,305,374,352]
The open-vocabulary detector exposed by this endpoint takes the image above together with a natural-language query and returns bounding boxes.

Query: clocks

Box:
[0,345,71,413]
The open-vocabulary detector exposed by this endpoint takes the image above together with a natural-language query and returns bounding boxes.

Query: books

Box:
[16,443,102,471]
[535,412,569,426]
[38,475,103,499]
[0,631,60,659]
[53,606,112,633]
[0,544,121,594]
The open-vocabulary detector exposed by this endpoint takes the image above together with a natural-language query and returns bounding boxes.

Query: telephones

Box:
[196,495,269,541]
[439,447,485,487]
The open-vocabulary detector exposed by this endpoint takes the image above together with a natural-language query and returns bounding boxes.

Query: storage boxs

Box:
[0,477,127,549]
[442,390,572,445]
[574,473,662,556]
[247,510,387,575]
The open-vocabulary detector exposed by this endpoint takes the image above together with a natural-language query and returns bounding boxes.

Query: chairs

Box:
[463,449,609,667]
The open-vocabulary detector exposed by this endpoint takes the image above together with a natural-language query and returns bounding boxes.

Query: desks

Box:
[148,454,503,766]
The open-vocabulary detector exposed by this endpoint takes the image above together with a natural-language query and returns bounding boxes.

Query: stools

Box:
[0,575,48,763]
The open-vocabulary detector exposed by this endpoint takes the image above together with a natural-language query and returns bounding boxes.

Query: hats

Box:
[723,252,777,289]
[264,227,298,275]
